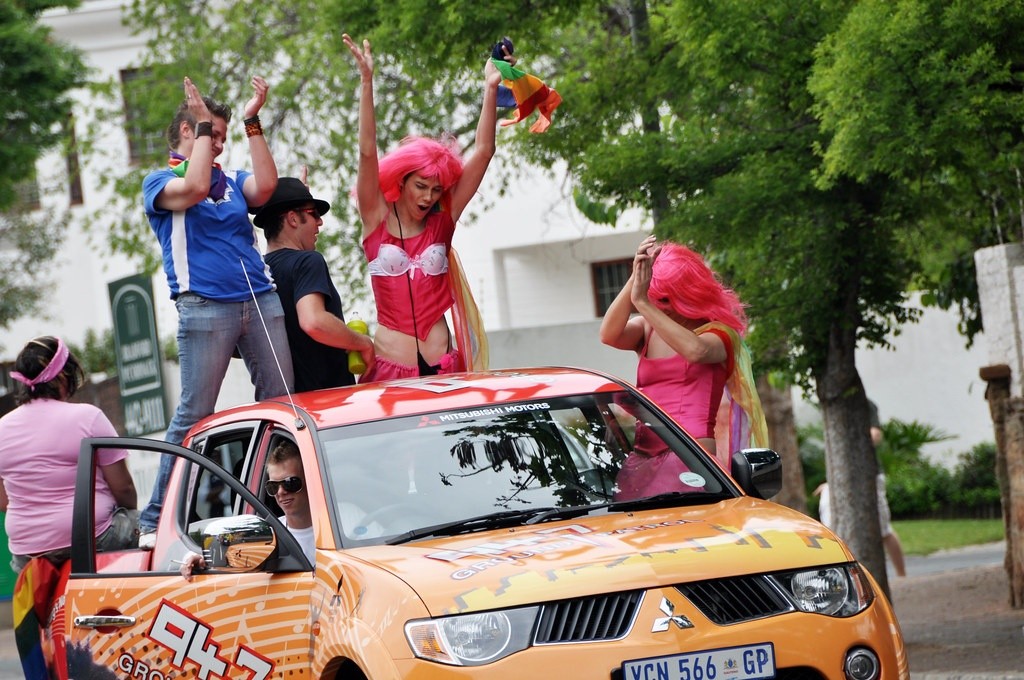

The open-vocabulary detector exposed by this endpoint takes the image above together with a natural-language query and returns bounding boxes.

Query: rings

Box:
[187,94,191,98]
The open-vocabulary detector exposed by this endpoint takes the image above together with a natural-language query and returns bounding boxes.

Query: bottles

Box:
[350,312,362,321]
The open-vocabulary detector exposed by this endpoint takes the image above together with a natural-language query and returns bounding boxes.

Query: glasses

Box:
[279,208,321,220]
[264,476,306,497]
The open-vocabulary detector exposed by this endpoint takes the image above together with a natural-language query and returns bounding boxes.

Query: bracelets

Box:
[243,116,263,137]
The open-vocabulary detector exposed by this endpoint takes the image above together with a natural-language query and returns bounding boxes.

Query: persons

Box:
[0,335,140,633]
[594,234,769,517]
[863,398,905,585]
[181,436,336,581]
[341,26,519,389]
[129,69,297,550]
[256,177,376,400]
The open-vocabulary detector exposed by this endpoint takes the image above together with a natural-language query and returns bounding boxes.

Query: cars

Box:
[41,365,915,680]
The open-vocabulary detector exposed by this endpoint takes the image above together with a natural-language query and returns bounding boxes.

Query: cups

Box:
[346,321,368,374]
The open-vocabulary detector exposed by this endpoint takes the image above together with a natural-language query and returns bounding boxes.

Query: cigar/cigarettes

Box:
[172,558,190,568]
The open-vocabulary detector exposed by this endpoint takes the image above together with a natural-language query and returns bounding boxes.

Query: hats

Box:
[252,177,330,228]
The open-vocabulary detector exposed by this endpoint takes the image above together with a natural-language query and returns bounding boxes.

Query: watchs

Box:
[194,121,213,140]
